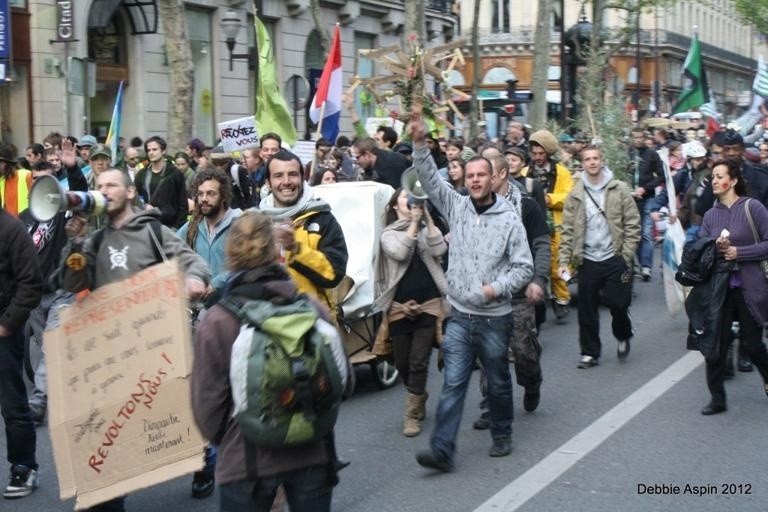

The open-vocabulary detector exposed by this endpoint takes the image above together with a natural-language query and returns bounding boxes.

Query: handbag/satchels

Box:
[760,259,768,279]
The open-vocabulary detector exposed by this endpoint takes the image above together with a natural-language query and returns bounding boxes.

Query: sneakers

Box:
[617,341,630,359]
[633,263,641,277]
[489,436,511,456]
[552,299,569,320]
[474,412,490,429]
[26,396,48,423]
[415,450,452,472]
[2,464,39,496]
[523,387,540,412]
[702,403,727,415]
[577,355,598,369]
[640,266,652,281]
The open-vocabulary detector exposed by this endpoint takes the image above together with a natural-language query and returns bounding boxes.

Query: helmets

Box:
[684,143,708,161]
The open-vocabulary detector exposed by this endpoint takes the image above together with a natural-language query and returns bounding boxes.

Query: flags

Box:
[753,50,767,96]
[310,22,341,144]
[60,80,124,190]
[670,27,710,114]
[253,17,298,150]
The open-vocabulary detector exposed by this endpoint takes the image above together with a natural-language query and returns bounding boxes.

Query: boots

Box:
[737,330,752,371]
[723,345,734,378]
[404,393,425,436]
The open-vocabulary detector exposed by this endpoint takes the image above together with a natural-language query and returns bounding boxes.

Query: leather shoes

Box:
[193,464,215,498]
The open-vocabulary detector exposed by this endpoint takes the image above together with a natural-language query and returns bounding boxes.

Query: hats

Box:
[722,128,743,145]
[504,147,525,161]
[745,147,760,163]
[90,144,112,161]
[211,147,233,160]
[559,134,574,142]
[394,142,413,153]
[0,144,17,163]
[74,135,97,150]
[528,130,558,155]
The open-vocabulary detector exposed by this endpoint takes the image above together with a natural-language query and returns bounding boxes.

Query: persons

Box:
[404,121,534,471]
[0,111,767,511]
[556,145,642,369]
[190,211,347,510]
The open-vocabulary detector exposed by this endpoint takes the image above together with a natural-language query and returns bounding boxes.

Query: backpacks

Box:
[217,291,351,496]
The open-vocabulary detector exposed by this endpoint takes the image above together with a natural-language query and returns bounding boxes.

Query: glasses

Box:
[353,154,363,161]
